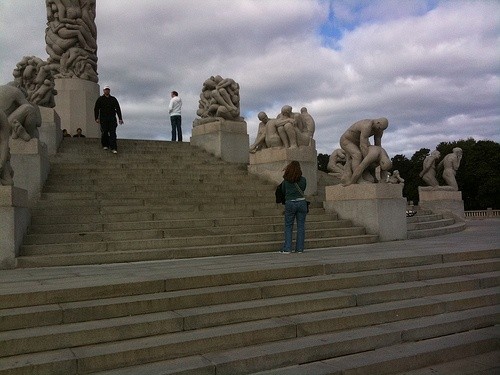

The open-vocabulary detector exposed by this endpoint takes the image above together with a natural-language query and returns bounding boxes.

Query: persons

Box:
[45,0,98,84]
[326,118,404,186]
[94,87,123,153]
[250,105,314,152]
[0,56,57,185]
[169,91,182,142]
[196,75,240,120]
[419,148,463,190]
[63,128,86,137]
[279,160,307,253]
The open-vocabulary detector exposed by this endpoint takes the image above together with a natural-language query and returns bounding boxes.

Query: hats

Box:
[103,85,110,90]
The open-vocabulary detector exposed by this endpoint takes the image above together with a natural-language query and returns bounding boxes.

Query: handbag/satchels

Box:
[305,200,311,213]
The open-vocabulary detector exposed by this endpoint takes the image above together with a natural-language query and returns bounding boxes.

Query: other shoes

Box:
[278,249,303,254]
[406,210,417,217]
[103,147,109,150]
[111,149,118,154]
